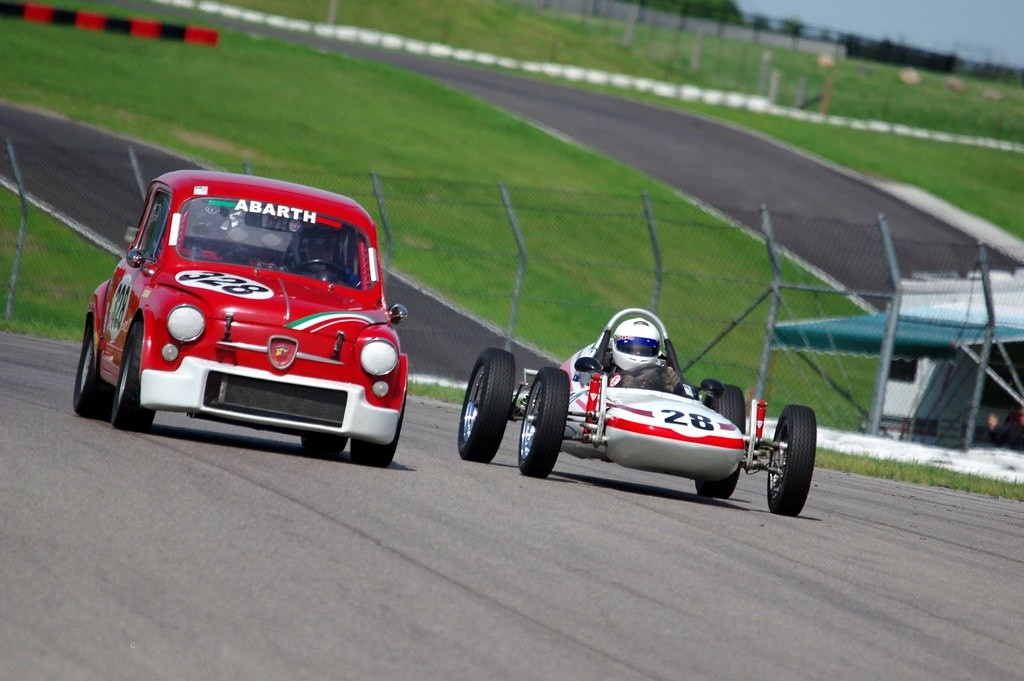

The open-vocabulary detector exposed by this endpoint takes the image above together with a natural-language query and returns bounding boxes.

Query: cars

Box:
[69,168,412,465]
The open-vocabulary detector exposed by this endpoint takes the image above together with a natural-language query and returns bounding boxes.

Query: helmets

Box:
[290,222,344,270]
[611,316,661,371]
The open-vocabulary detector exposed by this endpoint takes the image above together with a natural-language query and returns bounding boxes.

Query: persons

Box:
[277,229,363,290]
[607,325,687,396]
